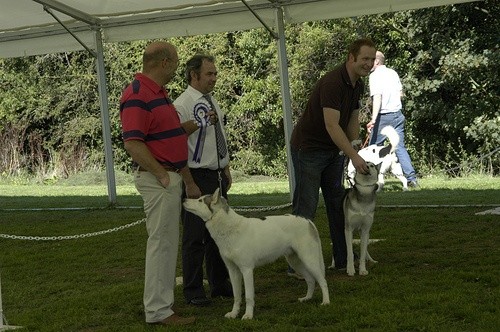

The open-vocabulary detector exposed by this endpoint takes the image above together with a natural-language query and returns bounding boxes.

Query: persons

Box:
[119,41,218,326]
[171,54,234,306]
[366,51,421,188]
[287,38,376,280]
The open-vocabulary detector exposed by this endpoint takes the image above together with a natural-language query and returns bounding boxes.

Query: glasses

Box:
[176,60,180,66]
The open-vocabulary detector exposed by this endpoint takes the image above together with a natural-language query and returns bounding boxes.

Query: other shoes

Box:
[333,258,375,272]
[288,270,305,279]
[150,304,196,326]
[401,180,420,188]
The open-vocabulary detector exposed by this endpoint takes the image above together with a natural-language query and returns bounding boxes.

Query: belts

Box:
[133,164,183,173]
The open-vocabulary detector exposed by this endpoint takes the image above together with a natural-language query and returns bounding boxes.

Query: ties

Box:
[202,94,228,159]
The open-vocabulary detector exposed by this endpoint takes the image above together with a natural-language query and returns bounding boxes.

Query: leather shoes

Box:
[191,298,210,306]
[211,280,235,299]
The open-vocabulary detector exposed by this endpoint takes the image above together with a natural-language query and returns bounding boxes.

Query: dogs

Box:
[341,159,383,277]
[343,124,410,193]
[181,186,332,321]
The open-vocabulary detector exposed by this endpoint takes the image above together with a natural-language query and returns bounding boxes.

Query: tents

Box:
[0,0,480,204]
